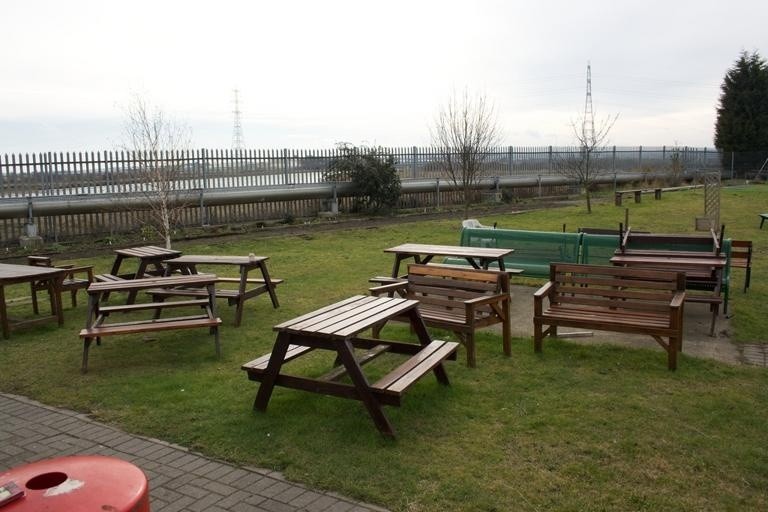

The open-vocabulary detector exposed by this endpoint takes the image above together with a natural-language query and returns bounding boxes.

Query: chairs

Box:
[28,255,89,326]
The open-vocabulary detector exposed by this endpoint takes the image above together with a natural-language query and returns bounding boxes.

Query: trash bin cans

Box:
[0,456,149,512]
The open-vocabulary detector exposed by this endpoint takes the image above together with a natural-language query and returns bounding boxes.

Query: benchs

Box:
[442,225,583,296]
[759,214,768,229]
[615,189,662,205]
[78,242,524,407]
[583,233,732,313]
[622,236,754,294]
[610,249,726,337]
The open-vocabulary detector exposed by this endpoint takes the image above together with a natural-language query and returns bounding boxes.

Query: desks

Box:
[253,294,452,447]
[0,264,71,338]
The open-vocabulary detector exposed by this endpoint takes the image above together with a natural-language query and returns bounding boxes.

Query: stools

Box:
[533,264,686,371]
